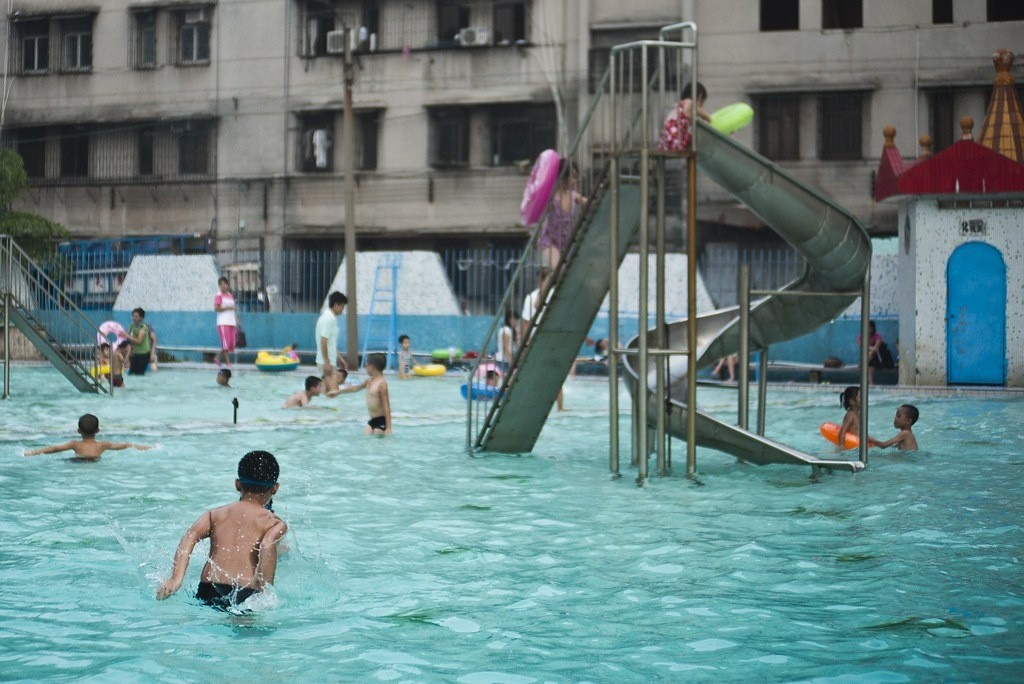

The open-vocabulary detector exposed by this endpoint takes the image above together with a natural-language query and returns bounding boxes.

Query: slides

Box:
[621,116,866,474]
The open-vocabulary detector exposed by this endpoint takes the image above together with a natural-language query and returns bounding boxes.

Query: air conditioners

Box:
[326,30,350,54]
[186,7,208,24]
[458,26,488,46]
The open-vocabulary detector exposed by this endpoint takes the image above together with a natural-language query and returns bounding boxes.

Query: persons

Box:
[213,277,238,367]
[327,353,391,435]
[659,80,712,151]
[217,368,231,388]
[156,451,287,608]
[858,321,899,388]
[398,335,425,380]
[485,370,500,391]
[520,268,595,411]
[712,353,739,382]
[867,404,920,451]
[315,291,348,393]
[93,307,158,387]
[25,413,155,458]
[495,309,517,377]
[839,386,861,450]
[597,339,622,362]
[282,376,323,408]
[535,158,586,310]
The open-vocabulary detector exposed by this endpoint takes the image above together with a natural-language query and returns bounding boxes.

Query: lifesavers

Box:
[687,102,754,138]
[519,147,560,226]
[255,351,301,370]
[413,364,445,376]
[459,384,506,401]
[821,422,873,450]
[96,320,126,352]
